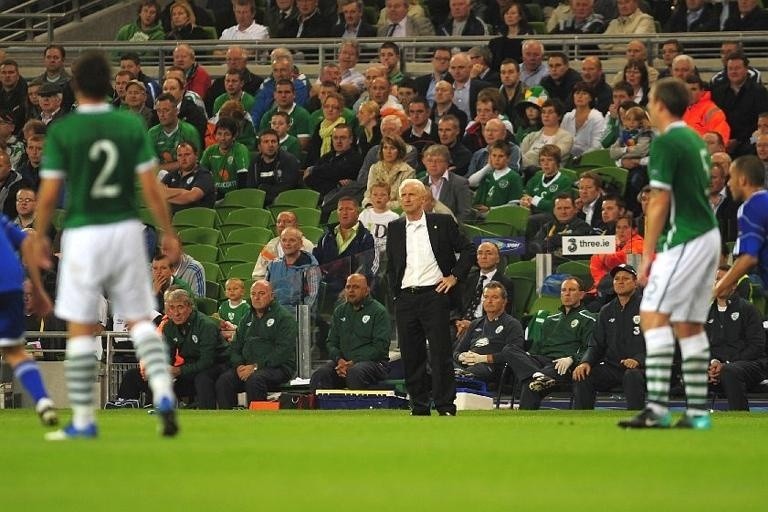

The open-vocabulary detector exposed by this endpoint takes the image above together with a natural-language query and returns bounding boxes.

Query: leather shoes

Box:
[439,411,455,417]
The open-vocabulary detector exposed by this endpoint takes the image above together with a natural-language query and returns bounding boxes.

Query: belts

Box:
[405,285,436,295]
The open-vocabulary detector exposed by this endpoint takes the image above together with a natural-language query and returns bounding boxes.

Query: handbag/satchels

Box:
[279,391,320,409]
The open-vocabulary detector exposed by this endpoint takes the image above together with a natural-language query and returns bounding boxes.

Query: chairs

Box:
[26,144,768,411]
[163,2,660,37]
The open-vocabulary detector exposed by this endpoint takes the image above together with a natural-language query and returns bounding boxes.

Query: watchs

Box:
[253,365,257,371]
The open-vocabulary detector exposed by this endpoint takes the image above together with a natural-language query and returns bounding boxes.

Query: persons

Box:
[117,289,218,409]
[113,1,768,65]
[716,155,768,298]
[385,179,473,416]
[0,207,60,429]
[572,264,646,410]
[613,75,727,434]
[452,281,524,390]
[704,264,768,410]
[310,273,392,395]
[0,41,768,360]
[502,276,596,410]
[30,48,183,444]
[215,279,298,409]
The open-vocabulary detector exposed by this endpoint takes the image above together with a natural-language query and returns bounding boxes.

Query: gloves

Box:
[465,352,488,366]
[458,350,469,365]
[552,357,573,376]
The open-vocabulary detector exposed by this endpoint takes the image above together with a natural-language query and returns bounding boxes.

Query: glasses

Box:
[15,198,35,202]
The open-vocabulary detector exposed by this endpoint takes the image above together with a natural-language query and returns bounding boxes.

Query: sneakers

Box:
[528,375,555,392]
[43,422,96,442]
[158,398,177,436]
[617,408,672,429]
[674,411,712,430]
[35,400,58,427]
[454,368,474,382]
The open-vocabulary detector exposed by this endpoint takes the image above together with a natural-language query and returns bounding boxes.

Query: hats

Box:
[37,82,62,96]
[125,80,146,92]
[515,85,549,117]
[610,263,637,277]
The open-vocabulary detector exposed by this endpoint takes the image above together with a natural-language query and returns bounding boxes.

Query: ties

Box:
[280,12,287,20]
[388,24,397,36]
[463,275,487,321]
